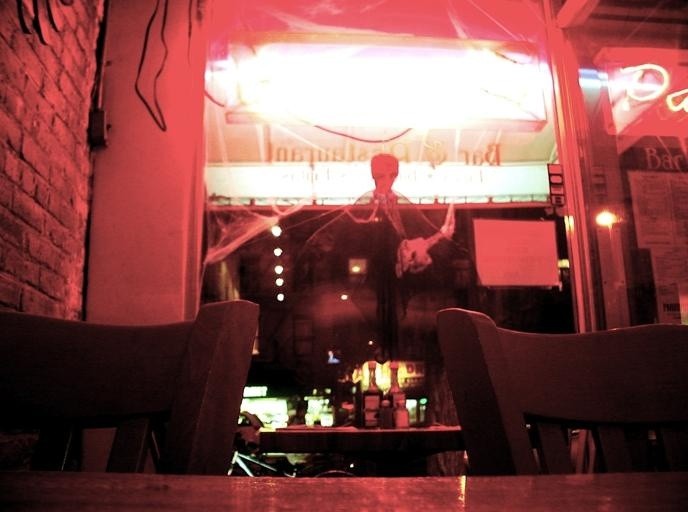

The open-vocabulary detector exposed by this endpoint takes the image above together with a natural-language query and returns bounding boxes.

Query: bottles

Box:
[310,389,321,425]
[319,388,334,428]
[283,396,302,426]
[417,398,428,423]
[335,361,410,430]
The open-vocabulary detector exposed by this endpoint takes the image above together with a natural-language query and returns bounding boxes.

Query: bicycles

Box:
[229,410,365,477]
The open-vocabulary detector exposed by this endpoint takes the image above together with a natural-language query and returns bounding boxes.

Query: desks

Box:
[0,467,688,512]
[257,421,463,477]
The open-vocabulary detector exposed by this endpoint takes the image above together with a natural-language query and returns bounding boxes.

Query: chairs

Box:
[431,305,687,478]
[1,292,262,476]
